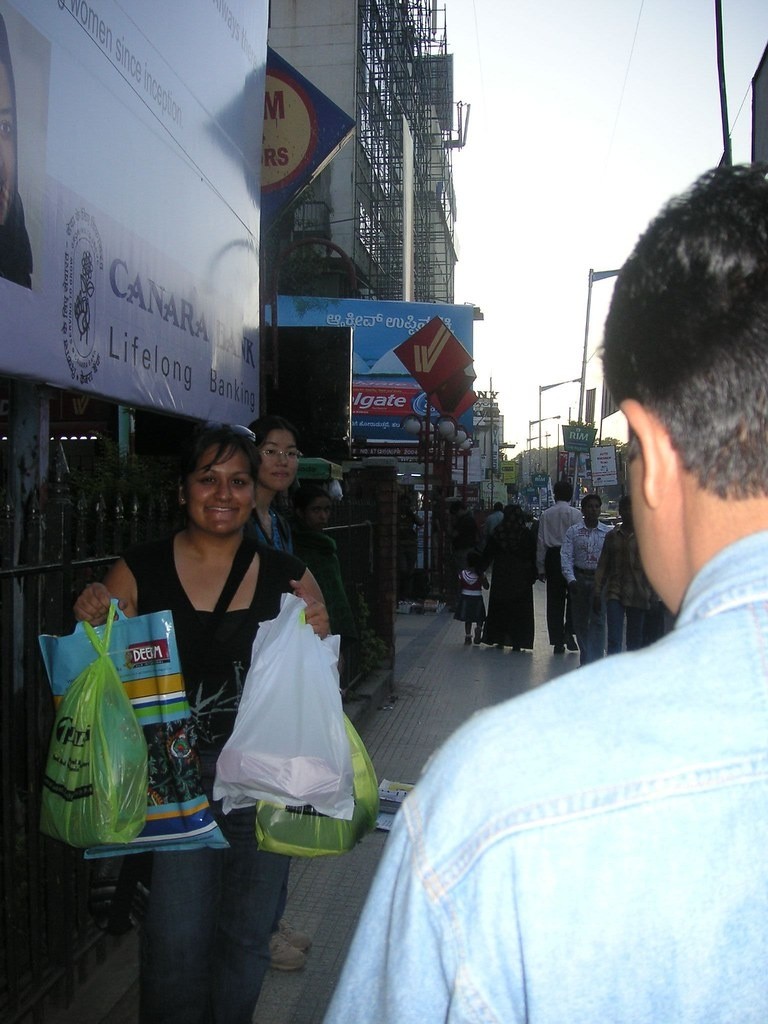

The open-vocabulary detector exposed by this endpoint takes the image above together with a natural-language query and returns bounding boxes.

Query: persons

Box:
[560,495,616,665]
[377,162,768,1024]
[453,551,489,646]
[478,504,539,652]
[73,421,333,1024]
[292,484,359,696]
[593,495,659,654]
[536,482,585,654]
[247,415,314,972]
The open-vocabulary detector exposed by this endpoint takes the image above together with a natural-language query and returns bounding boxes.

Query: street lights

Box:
[526,376,584,485]
[397,398,473,603]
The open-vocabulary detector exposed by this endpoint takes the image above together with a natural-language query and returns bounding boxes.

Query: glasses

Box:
[259,446,303,462]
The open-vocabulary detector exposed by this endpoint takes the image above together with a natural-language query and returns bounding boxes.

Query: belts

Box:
[573,565,597,576]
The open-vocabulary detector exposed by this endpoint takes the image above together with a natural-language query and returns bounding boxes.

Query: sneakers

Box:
[275,917,312,951]
[267,936,306,970]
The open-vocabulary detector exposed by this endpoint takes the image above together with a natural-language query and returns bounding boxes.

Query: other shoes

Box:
[465,635,472,644]
[474,637,481,646]
[567,642,578,651]
[512,646,520,651]
[554,645,566,653]
[496,644,505,648]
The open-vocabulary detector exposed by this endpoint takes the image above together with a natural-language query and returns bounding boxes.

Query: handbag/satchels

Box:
[211,590,380,854]
[38,597,231,860]
